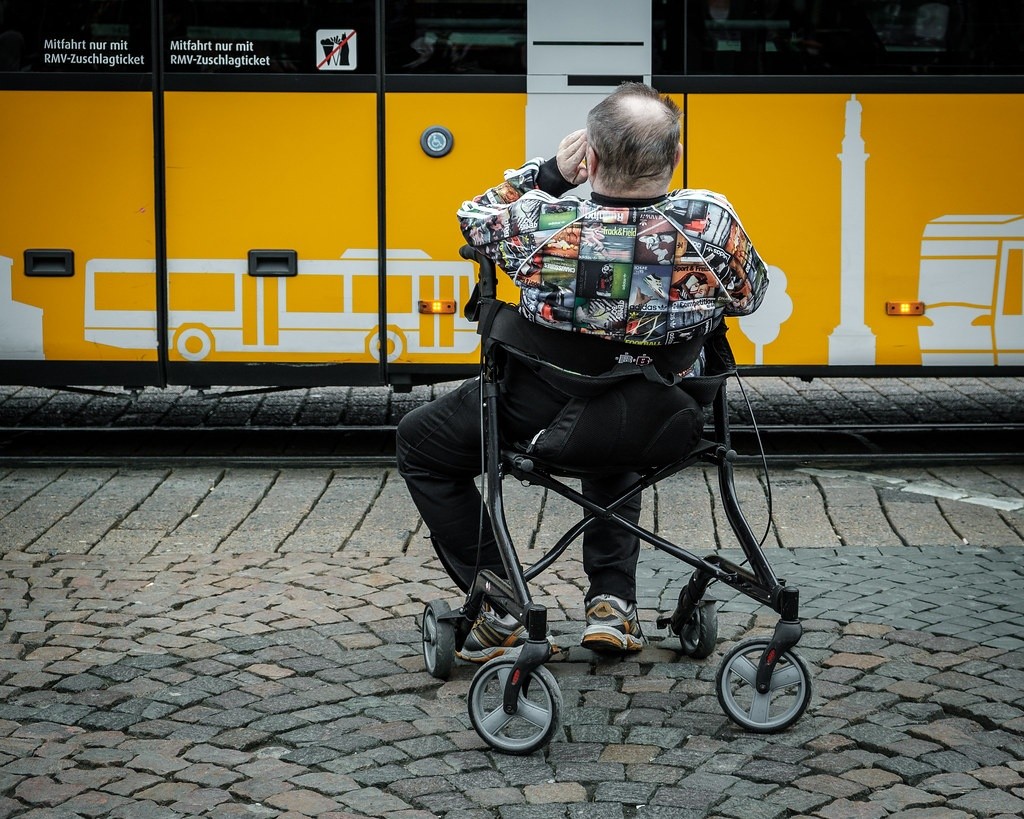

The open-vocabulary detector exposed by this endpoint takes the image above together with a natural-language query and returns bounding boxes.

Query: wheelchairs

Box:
[421,245,814,755]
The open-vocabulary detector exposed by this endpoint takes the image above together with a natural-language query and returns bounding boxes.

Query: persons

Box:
[396,82,769,661]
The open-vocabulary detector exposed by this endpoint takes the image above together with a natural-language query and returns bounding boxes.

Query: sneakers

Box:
[580,596,648,655]
[454,601,560,664]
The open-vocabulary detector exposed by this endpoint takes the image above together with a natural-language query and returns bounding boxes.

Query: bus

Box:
[0,0,1024,394]
